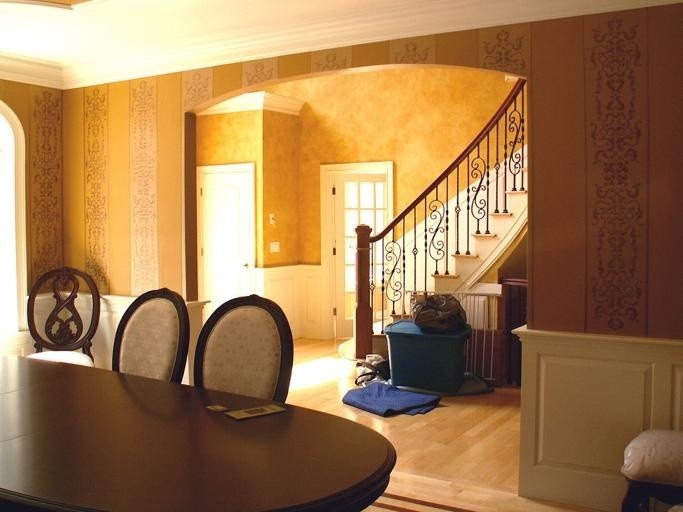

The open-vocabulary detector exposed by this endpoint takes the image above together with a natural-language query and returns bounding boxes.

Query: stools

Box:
[619,429,683,512]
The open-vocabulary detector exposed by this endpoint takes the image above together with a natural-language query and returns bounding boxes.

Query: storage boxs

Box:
[382,320,473,394]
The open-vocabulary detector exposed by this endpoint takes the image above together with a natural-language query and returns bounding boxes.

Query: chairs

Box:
[193,294,293,403]
[112,288,190,384]
[27,266,99,368]
